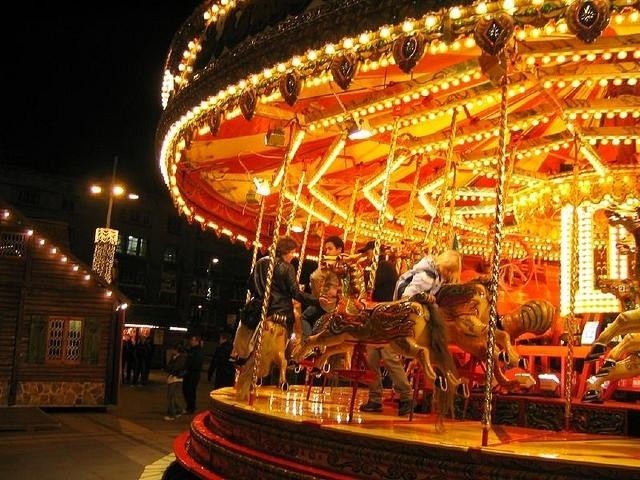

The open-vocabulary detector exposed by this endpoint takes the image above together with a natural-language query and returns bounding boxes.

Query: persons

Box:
[121,329,236,421]
[394,250,461,302]
[300,236,344,336]
[357,239,419,415]
[242,237,321,359]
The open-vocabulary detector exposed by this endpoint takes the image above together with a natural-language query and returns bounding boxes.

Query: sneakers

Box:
[359,400,383,410]
[399,398,418,415]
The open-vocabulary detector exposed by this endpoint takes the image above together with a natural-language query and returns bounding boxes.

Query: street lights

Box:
[84,155,124,228]
[206,258,220,312]
